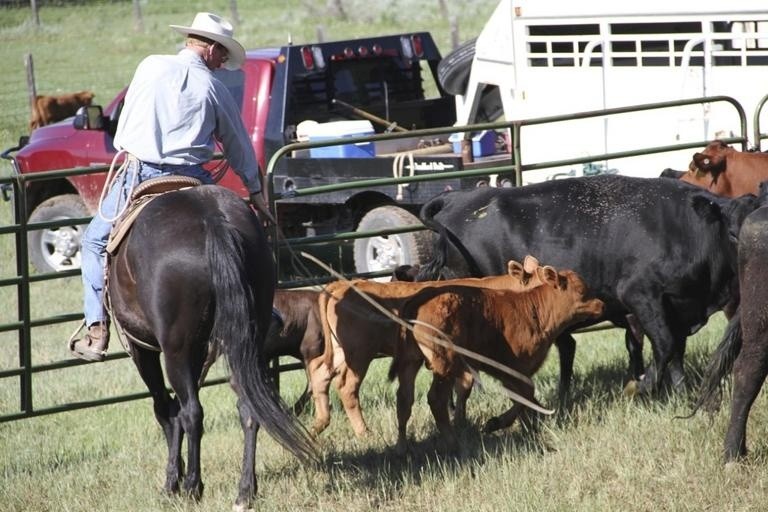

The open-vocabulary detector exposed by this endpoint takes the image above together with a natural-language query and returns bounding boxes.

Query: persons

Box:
[68,11,278,363]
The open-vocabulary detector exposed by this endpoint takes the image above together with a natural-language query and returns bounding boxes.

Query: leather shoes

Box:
[73,324,110,363]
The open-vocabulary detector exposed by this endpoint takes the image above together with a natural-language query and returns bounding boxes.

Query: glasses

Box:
[220,47,231,63]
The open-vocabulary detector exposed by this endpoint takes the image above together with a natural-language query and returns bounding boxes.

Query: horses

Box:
[106,183,332,512]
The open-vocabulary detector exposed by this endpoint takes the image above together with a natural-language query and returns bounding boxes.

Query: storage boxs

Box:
[310,119,376,159]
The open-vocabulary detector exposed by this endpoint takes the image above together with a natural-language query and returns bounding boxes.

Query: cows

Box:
[397,173,768,420]
[310,255,550,438]
[25,90,95,129]
[392,266,606,455]
[680,138,768,476]
[262,287,334,411]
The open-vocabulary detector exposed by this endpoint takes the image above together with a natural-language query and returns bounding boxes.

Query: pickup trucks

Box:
[3,27,514,287]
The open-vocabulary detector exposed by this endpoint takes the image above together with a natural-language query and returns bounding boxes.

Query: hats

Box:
[169,12,246,72]
